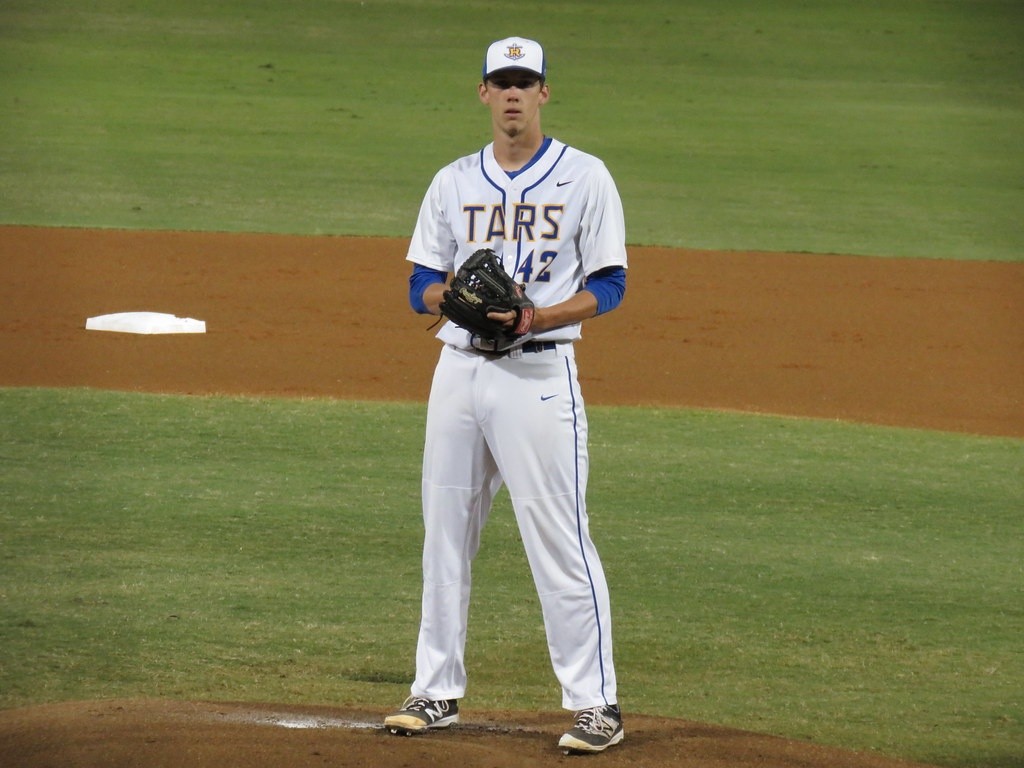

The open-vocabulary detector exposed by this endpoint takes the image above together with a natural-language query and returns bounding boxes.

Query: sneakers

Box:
[558,704,624,752]
[384,696,459,736]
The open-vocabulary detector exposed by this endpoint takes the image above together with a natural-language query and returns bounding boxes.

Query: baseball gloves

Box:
[439,248,536,343]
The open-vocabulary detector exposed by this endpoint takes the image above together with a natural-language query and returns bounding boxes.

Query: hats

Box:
[482,36,546,80]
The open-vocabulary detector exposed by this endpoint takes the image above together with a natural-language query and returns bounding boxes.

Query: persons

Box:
[383,36,627,752]
